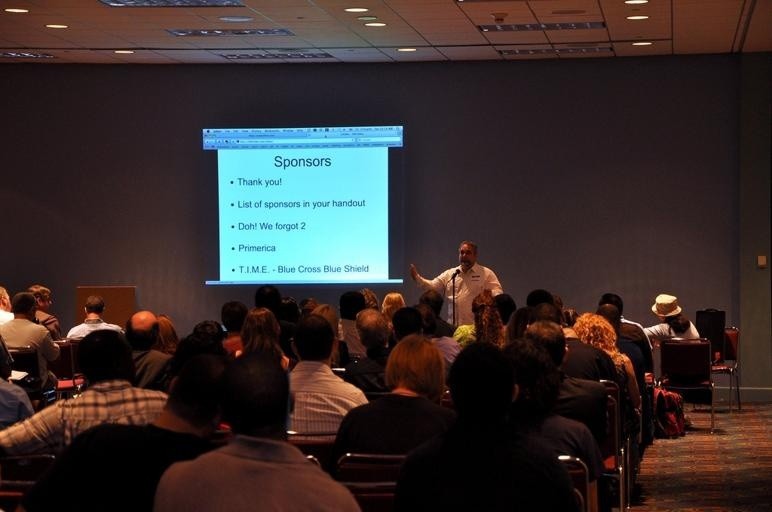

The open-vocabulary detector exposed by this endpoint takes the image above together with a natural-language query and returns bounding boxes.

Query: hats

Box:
[651,294,682,316]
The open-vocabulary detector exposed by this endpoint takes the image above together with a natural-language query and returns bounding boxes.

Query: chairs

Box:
[710,325,742,415]
[659,336,717,436]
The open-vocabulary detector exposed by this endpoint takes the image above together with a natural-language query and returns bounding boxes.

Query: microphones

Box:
[448,269,460,282]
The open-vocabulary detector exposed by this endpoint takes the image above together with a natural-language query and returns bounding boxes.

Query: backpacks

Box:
[653,387,685,437]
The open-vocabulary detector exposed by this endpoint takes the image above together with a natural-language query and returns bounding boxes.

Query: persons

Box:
[644,294,701,343]
[410,241,504,327]
[0,289,655,512]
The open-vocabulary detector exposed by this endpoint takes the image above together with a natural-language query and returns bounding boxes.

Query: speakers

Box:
[696,309,726,362]
